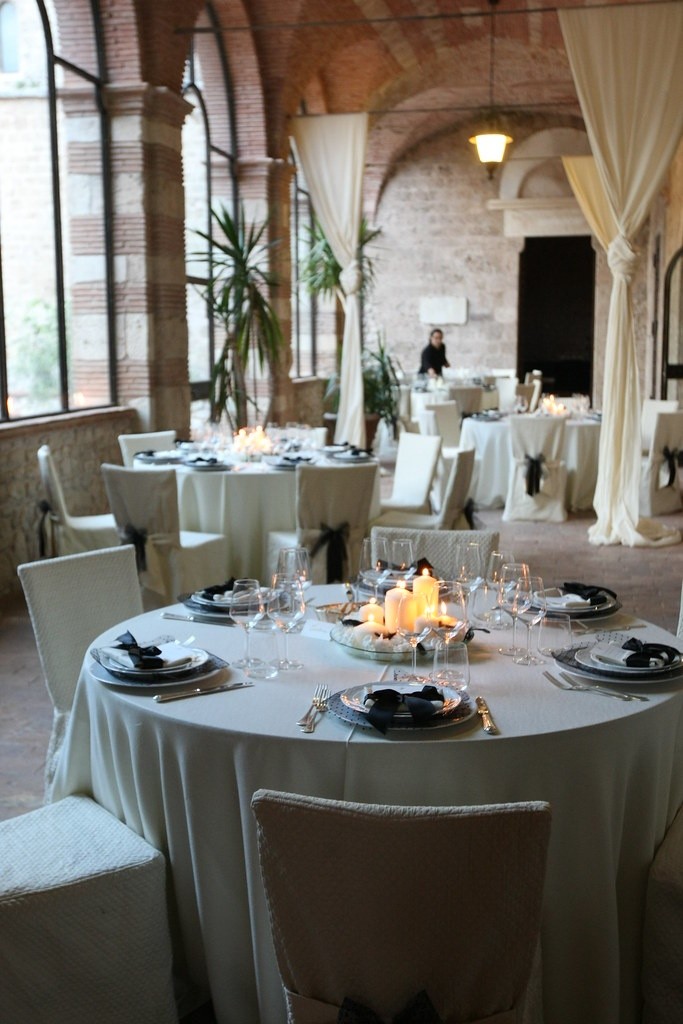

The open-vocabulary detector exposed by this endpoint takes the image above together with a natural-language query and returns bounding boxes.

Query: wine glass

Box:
[229,536,547,686]
[177,423,327,455]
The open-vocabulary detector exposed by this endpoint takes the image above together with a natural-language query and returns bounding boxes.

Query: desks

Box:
[456,410,602,516]
[46,583,683,1024]
[407,387,448,437]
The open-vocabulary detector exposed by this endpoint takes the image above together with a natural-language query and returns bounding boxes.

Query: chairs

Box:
[369,525,501,582]
[246,787,552,1024]
[266,424,329,446]
[15,543,146,802]
[376,370,683,529]
[99,461,232,613]
[0,789,185,1024]
[636,802,683,1024]
[117,429,177,468]
[264,461,378,586]
[33,443,124,562]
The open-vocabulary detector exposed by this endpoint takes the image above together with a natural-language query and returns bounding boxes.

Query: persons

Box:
[420,328,450,379]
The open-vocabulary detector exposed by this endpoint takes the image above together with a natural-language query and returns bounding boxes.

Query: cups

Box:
[433,642,471,690]
[537,613,572,656]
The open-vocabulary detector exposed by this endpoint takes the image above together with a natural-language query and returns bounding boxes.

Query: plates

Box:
[135,443,374,472]
[99,649,209,680]
[340,682,462,718]
[88,661,223,687]
[508,588,622,622]
[555,647,683,683]
[178,590,292,619]
[574,647,682,673]
[325,685,478,731]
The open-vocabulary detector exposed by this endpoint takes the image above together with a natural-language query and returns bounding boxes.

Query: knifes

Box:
[154,683,254,701]
[475,696,496,733]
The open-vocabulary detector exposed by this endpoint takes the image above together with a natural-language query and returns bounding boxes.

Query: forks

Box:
[297,685,332,732]
[543,671,650,701]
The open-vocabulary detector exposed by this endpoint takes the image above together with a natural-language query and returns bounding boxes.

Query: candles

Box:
[414,604,439,633]
[384,581,412,633]
[358,597,383,624]
[412,567,439,634]
[438,601,459,628]
[357,615,389,639]
[232,426,274,461]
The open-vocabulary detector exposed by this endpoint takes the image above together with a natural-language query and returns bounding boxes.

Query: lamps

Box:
[464,0,516,181]
